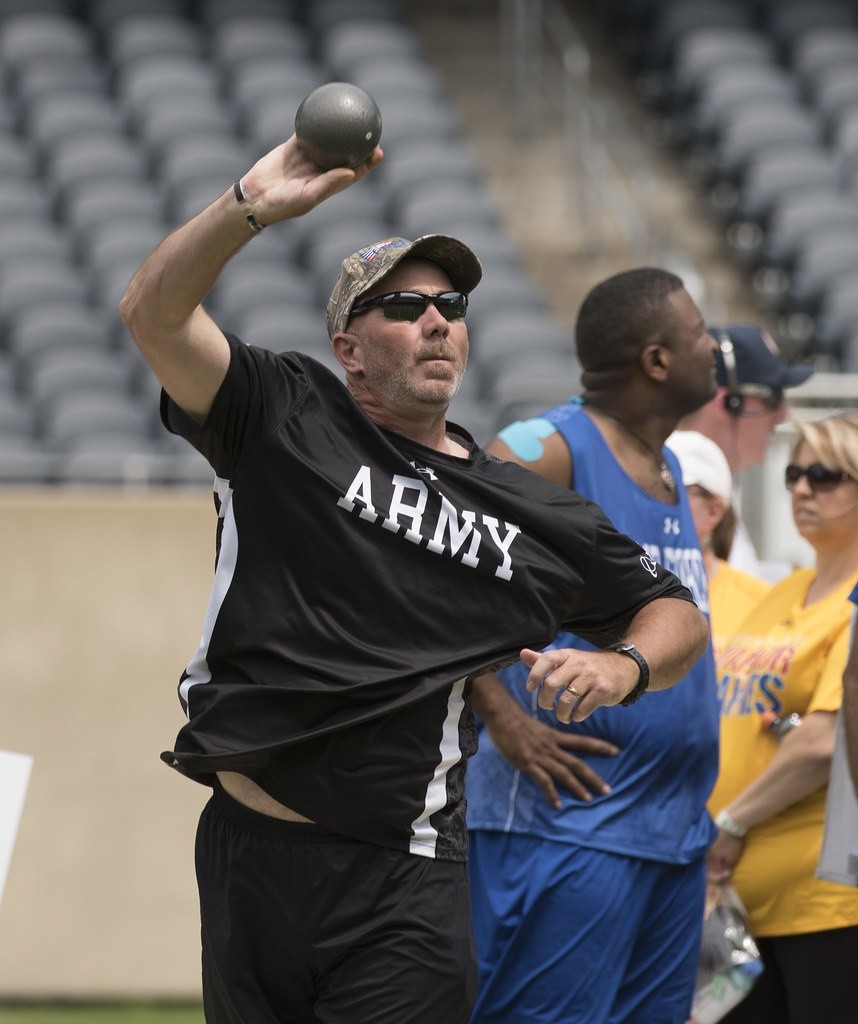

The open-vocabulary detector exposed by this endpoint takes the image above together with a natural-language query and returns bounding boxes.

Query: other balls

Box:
[292,80,385,172]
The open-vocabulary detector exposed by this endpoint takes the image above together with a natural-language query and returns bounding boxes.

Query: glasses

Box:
[742,387,782,418]
[785,463,855,491]
[350,291,468,322]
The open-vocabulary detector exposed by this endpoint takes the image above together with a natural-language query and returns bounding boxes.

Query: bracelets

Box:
[716,811,746,838]
[609,643,649,706]
[233,180,263,233]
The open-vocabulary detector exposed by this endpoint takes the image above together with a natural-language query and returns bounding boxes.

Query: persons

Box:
[120,130,709,1023]
[470,268,720,1024]
[666,326,858,1024]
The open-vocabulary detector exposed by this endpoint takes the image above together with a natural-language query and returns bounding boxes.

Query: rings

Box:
[566,687,580,698]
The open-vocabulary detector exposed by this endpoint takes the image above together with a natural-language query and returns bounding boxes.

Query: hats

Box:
[664,430,732,507]
[707,325,814,390]
[326,234,483,345]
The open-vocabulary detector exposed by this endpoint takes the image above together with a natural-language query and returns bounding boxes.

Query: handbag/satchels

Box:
[684,880,765,1024]
